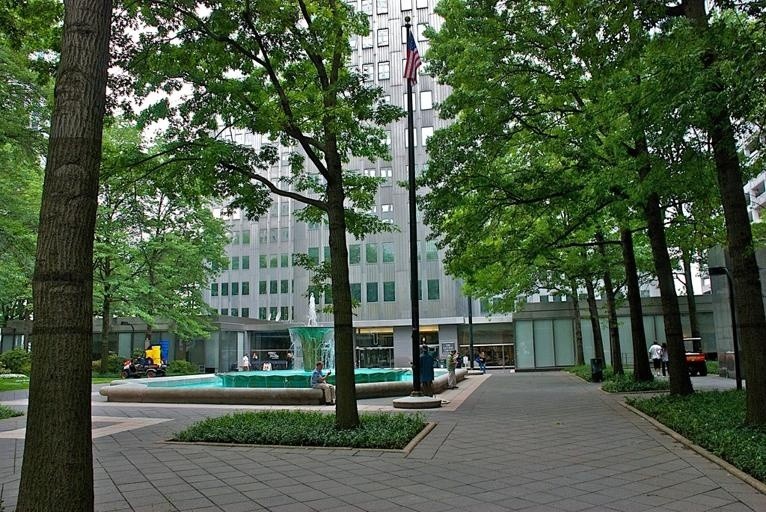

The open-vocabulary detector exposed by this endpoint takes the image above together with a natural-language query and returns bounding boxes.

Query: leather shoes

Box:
[327,402,333,405]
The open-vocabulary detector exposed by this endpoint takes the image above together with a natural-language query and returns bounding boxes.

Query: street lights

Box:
[120,321,133,358]
[708,264,741,391]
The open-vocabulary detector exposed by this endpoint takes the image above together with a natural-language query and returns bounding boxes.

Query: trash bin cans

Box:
[590,358,603,382]
[438,359,446,368]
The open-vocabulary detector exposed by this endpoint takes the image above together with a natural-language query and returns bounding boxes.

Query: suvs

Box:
[120,358,166,379]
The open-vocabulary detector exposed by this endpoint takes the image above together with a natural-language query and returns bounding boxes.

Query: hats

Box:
[422,344,429,352]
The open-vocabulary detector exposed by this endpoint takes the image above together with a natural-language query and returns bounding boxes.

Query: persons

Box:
[421,345,434,397]
[446,349,458,389]
[455,352,462,368]
[480,351,486,373]
[242,352,249,371]
[310,361,335,405]
[658,342,671,377]
[648,340,662,377]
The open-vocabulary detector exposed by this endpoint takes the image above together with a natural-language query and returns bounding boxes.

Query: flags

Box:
[403,30,422,87]
